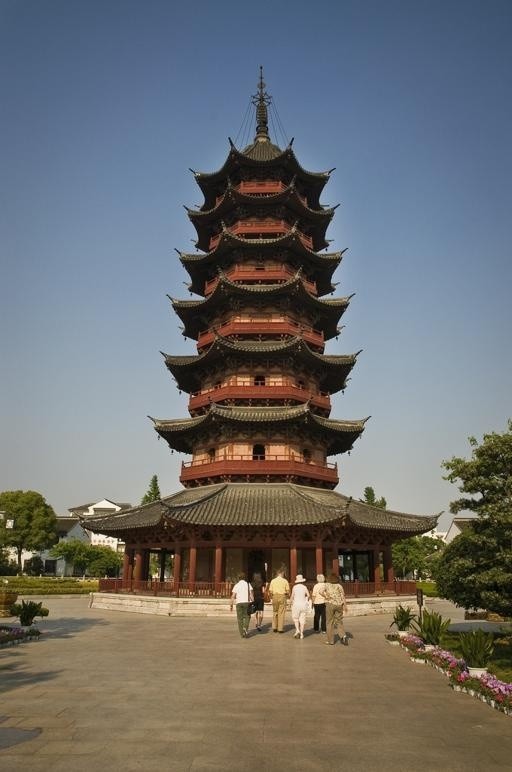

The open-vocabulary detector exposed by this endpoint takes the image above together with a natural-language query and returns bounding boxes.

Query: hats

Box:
[327,575,339,582]
[294,574,306,583]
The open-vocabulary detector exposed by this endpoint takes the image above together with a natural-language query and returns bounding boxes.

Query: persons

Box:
[268,568,291,633]
[319,575,349,646]
[289,574,310,639]
[230,571,255,639]
[250,571,268,631]
[311,574,332,634]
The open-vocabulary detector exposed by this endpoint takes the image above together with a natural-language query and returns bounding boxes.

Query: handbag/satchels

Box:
[247,603,257,615]
[263,591,270,602]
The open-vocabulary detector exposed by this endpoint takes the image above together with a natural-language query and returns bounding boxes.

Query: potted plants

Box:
[6,599,43,641]
[384,604,418,645]
[456,626,496,680]
[409,607,451,652]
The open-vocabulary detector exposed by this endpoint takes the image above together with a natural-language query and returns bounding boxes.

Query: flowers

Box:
[400,634,512,715]
[0,625,26,647]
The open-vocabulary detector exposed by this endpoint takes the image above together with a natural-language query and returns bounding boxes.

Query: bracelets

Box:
[230,607,232,608]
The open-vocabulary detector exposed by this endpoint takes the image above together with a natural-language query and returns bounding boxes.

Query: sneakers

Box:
[240,624,262,638]
[314,629,348,645]
[272,627,304,640]
